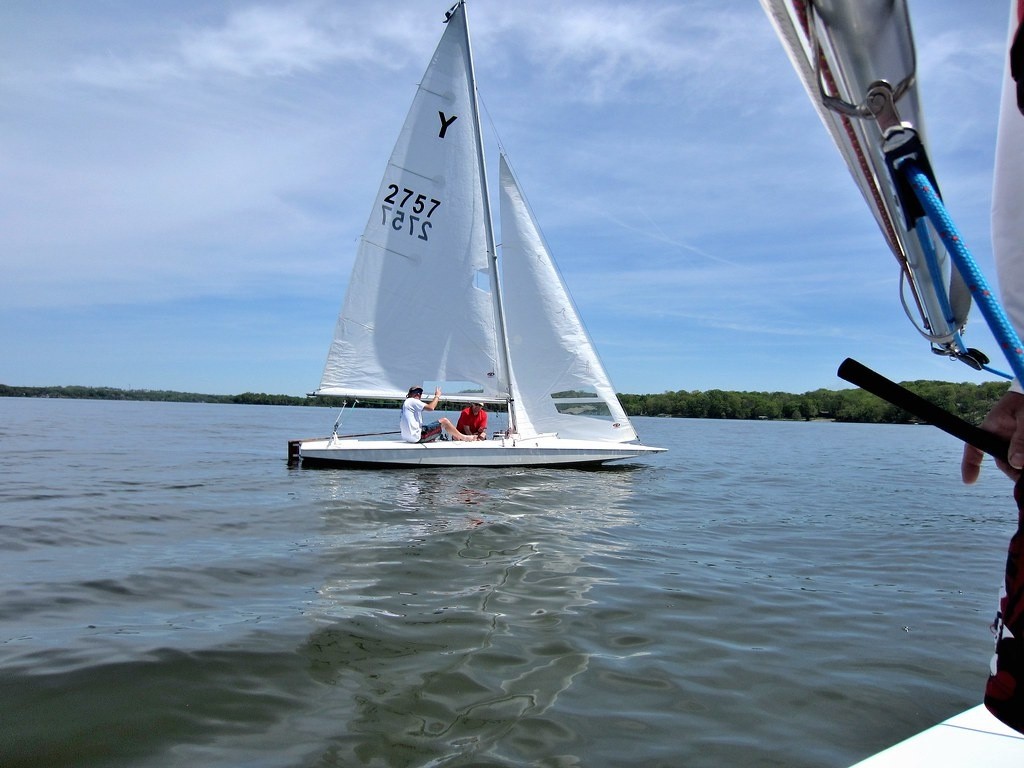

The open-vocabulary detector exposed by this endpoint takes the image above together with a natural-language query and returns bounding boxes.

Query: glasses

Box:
[473,403,482,407]
[412,390,422,394]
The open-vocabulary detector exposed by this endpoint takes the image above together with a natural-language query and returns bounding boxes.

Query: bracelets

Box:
[435,396,439,399]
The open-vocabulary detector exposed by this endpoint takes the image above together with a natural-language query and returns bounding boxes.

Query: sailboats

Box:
[287,0,668,467]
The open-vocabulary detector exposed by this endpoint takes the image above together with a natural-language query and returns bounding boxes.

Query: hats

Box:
[469,402,484,407]
[409,386,424,392]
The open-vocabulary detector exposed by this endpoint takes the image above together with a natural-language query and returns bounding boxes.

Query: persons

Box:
[961,376,1024,733]
[456,403,488,441]
[400,387,478,443]
